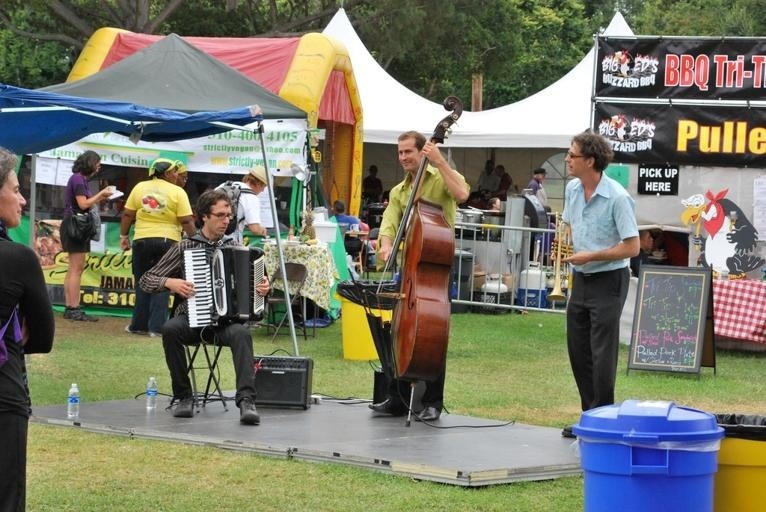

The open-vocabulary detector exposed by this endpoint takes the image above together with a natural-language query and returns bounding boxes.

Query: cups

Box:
[350,224,360,232]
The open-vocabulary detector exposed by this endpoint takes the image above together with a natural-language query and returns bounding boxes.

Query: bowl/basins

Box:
[312,223,338,243]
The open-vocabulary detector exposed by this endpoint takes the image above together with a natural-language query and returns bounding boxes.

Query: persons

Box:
[550,132,641,437]
[121,157,196,335]
[0,146,55,512]
[139,189,270,424]
[330,159,556,273]
[221,166,271,246]
[630,230,654,278]
[59,150,114,323]
[368,130,471,422]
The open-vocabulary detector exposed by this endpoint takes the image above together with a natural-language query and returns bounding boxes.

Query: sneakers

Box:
[125,325,157,337]
[63,309,99,321]
[174,398,194,417]
[240,398,260,422]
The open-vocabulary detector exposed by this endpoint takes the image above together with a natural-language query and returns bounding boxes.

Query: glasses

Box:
[569,152,588,159]
[210,212,234,220]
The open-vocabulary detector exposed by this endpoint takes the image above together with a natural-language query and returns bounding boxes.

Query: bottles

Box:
[287,225,294,241]
[520,262,545,297]
[480,274,508,304]
[146,376,158,410]
[67,383,81,417]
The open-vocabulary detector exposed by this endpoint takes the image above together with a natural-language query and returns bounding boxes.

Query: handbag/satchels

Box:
[66,204,101,245]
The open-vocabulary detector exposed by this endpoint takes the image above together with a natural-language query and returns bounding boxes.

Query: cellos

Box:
[390,96,463,381]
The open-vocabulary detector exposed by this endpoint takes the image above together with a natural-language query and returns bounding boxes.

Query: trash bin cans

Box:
[570,400,765,510]
[335,280,399,361]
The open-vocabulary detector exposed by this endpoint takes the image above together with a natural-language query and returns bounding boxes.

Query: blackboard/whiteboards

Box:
[626,267,710,372]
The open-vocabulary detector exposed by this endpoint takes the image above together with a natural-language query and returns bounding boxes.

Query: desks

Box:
[345,230,370,279]
[263,240,328,337]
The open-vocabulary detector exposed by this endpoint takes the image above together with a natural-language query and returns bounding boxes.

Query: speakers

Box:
[253,355,314,410]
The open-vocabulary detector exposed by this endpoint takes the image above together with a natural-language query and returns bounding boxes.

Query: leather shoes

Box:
[369,396,413,415]
[416,407,441,421]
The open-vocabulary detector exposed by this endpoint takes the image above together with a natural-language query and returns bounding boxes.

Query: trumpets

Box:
[546,211,575,301]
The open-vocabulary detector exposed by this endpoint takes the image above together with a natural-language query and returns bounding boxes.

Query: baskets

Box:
[469,288,548,314]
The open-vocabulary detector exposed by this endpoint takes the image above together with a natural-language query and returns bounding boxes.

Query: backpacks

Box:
[215,181,255,235]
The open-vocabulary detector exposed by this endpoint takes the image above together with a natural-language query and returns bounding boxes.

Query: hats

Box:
[247,164,268,186]
[149,158,188,182]
[533,168,548,174]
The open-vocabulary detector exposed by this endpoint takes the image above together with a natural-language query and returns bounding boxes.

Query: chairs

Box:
[362,228,397,280]
[266,263,308,342]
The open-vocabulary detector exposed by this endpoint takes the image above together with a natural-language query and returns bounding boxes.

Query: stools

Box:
[165,342,229,412]
